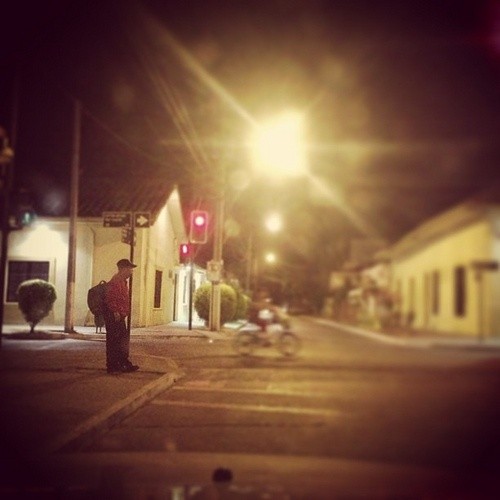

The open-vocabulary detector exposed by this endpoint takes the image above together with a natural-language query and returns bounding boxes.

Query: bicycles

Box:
[228,324,301,359]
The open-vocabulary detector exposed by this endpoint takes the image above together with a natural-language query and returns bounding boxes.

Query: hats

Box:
[116,258,137,268]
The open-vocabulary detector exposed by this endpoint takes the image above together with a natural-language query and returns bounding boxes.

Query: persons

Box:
[102,259,139,373]
[248,288,279,347]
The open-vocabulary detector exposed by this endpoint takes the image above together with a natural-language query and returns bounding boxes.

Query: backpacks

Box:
[87,280,107,314]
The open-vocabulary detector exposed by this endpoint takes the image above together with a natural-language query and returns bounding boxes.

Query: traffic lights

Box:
[189,210,208,245]
[180,243,192,257]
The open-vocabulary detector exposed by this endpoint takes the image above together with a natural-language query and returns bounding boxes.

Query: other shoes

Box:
[108,365,138,373]
